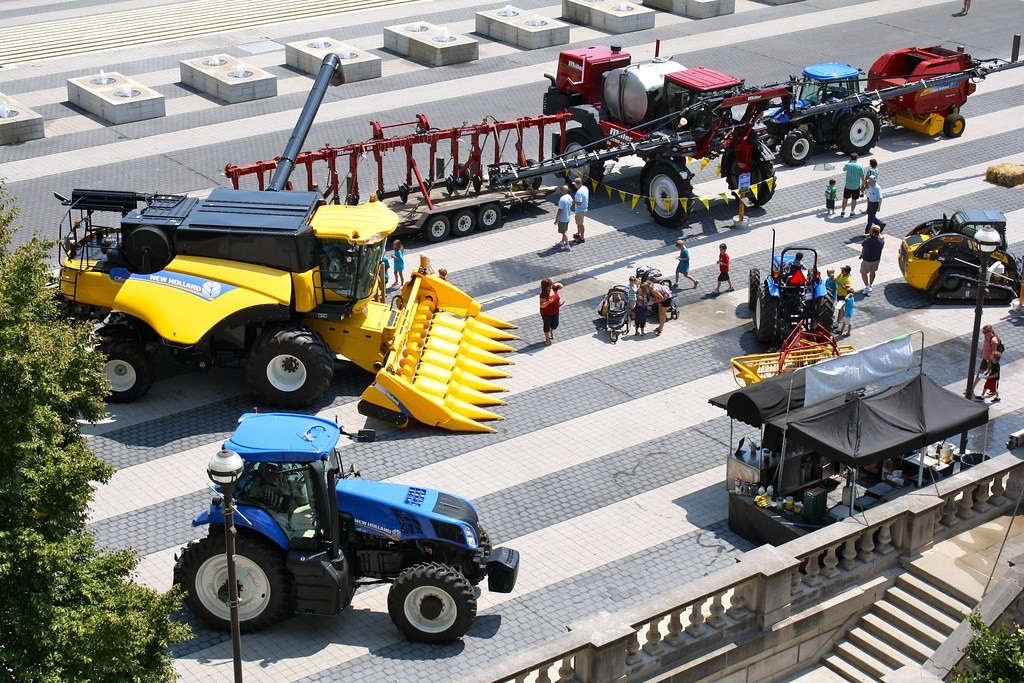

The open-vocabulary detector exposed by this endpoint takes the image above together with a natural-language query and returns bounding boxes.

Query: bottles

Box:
[734,476,804,514]
[800,465,811,482]
[764,450,770,460]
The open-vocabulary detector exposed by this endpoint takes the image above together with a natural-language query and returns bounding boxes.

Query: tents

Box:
[773,375,988,518]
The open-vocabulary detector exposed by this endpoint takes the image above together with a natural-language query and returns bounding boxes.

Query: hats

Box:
[840,265,851,273]
[867,175,876,182]
[572,178,581,185]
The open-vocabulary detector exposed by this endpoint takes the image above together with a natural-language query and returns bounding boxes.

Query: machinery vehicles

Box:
[171,406,521,647]
[747,227,835,346]
[542,38,794,230]
[762,33,1024,166]
[49,50,519,437]
[897,208,1023,307]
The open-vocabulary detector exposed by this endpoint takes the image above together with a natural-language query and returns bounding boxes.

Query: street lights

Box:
[205,443,246,683]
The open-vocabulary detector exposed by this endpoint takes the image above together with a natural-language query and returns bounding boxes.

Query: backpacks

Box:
[825,187,834,200]
[990,336,1004,354]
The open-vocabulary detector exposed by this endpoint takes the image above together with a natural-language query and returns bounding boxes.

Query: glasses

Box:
[982,331,988,334]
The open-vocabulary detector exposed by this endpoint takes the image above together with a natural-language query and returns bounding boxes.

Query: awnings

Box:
[711,368,806,427]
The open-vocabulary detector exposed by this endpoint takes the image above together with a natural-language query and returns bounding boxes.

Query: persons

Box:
[673,240,699,287]
[554,184,572,251]
[964,324,1002,401]
[858,225,884,294]
[641,281,671,336]
[633,296,646,336]
[824,268,838,300]
[626,276,647,308]
[391,240,405,285]
[540,279,563,346]
[833,265,851,329]
[712,243,735,295]
[572,178,589,244]
[379,256,390,284]
[836,288,854,337]
[783,253,806,278]
[962,0,971,13]
[827,153,887,234]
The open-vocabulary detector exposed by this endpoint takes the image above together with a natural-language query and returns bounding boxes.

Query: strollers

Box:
[596,265,680,343]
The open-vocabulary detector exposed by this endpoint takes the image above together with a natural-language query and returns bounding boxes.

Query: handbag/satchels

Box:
[559,298,565,307]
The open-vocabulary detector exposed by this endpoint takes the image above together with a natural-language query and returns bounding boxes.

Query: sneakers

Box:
[862,285,873,294]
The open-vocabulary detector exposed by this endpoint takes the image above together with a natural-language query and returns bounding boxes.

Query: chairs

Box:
[829,482,902,522]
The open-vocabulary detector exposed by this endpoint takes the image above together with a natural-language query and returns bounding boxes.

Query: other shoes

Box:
[841,212,845,217]
[693,280,698,289]
[635,333,645,336]
[987,391,998,395]
[845,333,850,337]
[399,286,403,290]
[880,224,886,233]
[550,329,553,338]
[833,320,841,327]
[712,291,720,296]
[573,234,585,243]
[850,212,855,217]
[555,241,571,252]
[392,283,398,286]
[653,328,663,336]
[726,287,734,292]
[991,398,1000,403]
[974,396,984,402]
[545,341,551,345]
[673,283,678,287]
[835,329,842,335]
[962,390,974,394]
